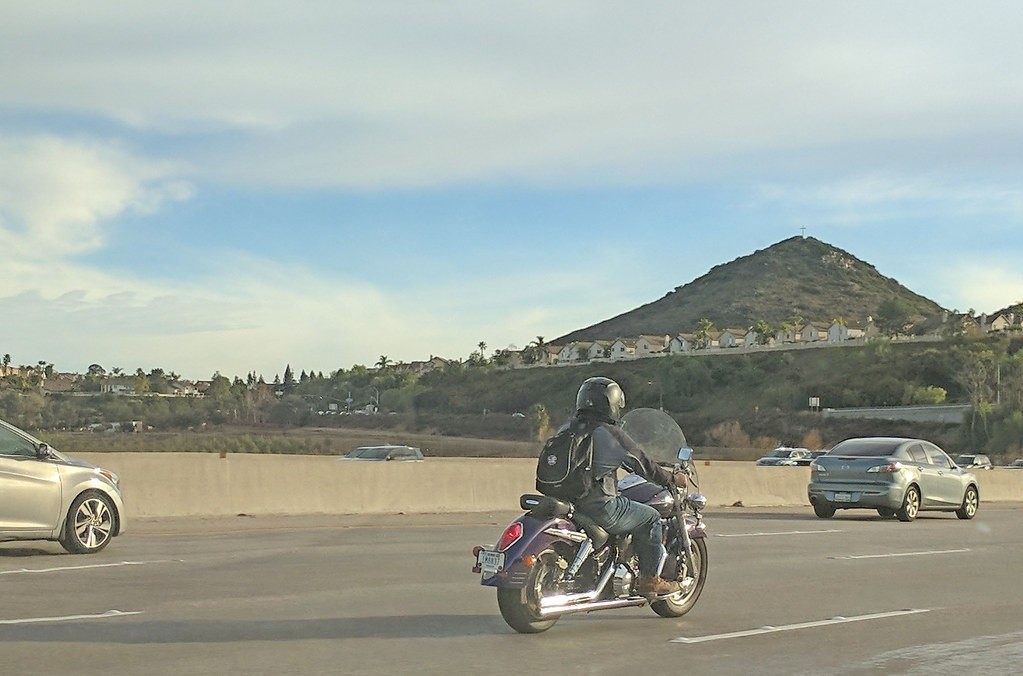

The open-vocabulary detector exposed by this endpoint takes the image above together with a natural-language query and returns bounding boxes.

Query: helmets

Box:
[576,376,621,421]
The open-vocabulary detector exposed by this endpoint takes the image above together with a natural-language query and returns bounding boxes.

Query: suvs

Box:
[348,444,425,462]
[954,454,992,471]
[756,446,810,468]
[334,447,380,459]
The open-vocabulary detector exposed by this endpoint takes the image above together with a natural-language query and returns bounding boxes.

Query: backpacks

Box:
[535,419,603,500]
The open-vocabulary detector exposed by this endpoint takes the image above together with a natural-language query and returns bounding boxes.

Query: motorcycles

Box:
[470,406,709,633]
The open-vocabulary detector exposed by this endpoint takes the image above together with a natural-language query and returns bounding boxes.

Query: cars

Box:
[807,436,981,521]
[0,419,128,553]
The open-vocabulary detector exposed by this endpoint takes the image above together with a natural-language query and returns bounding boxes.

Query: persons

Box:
[545,376,682,599]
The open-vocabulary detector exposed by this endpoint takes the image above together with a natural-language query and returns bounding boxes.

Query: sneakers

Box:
[639,576,678,597]
